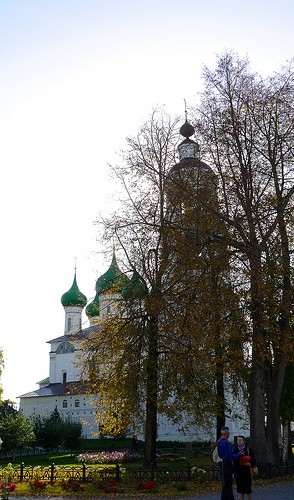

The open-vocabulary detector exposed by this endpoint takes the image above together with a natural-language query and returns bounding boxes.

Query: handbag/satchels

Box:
[251,467,258,475]
[209,443,223,463]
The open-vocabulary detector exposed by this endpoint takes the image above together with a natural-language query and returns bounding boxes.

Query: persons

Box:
[217,427,245,500]
[233,435,257,500]
